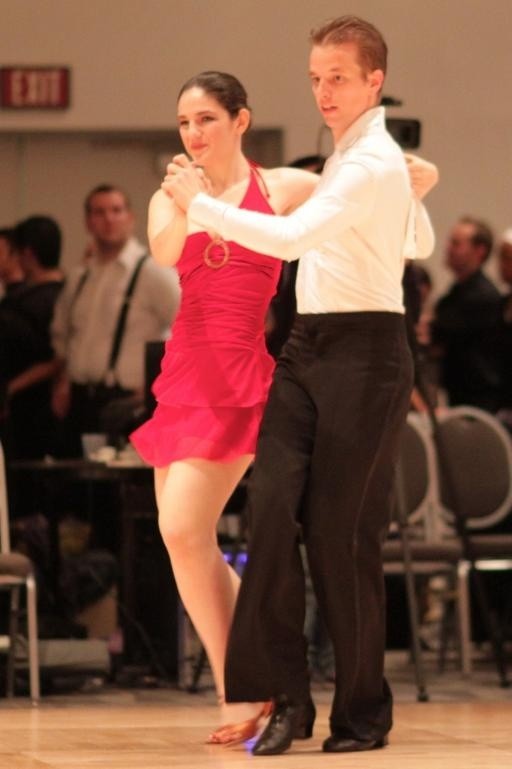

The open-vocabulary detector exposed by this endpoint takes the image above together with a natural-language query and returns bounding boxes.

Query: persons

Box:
[0,185,183,541]
[129,71,439,748]
[400,217,511,425]
[159,15,436,756]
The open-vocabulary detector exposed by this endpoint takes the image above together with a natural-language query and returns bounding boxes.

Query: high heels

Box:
[208,697,275,747]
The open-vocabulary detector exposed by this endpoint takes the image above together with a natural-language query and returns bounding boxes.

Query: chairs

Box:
[380,405,511,702]
[0,443,39,706]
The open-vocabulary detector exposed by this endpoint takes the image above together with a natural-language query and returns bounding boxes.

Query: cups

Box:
[80,431,107,469]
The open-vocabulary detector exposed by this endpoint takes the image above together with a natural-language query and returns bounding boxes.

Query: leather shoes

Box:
[251,693,316,755]
[322,733,389,750]
